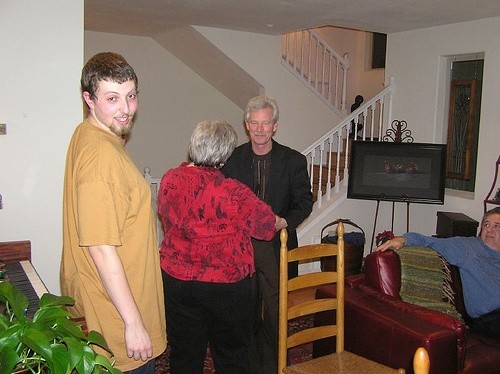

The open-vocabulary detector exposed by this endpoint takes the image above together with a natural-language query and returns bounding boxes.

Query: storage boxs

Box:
[436,211,479,239]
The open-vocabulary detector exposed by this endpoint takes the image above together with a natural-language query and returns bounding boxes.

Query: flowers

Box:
[376,230,394,248]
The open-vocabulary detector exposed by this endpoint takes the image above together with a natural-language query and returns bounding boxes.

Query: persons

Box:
[220,96,313,374]
[60,52,168,374]
[157,119,278,374]
[373,206,500,374]
[349,95,364,139]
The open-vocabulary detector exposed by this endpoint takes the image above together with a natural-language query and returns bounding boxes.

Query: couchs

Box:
[312,249,500,373]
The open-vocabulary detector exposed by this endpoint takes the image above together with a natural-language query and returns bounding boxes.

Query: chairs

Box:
[278,221,406,374]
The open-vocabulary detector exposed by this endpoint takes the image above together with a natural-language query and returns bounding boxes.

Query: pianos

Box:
[0,240,87,374]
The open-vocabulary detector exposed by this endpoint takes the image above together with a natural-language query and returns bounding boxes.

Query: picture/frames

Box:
[444,79,476,180]
